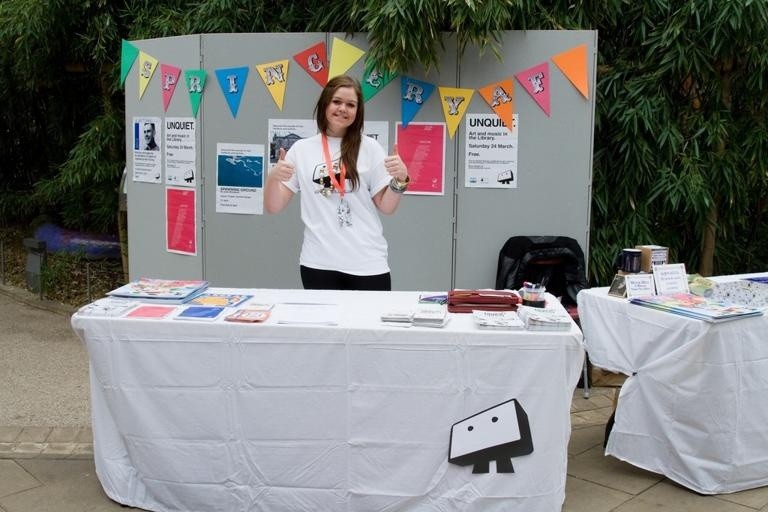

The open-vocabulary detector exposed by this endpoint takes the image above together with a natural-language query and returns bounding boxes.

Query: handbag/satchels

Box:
[541,265,578,305]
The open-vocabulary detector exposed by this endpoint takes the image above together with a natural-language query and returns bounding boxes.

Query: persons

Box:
[143,123,160,151]
[264,75,409,291]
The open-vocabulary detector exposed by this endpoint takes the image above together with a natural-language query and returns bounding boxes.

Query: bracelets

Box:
[389,176,409,193]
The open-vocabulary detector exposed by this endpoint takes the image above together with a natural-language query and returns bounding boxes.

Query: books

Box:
[473,304,572,334]
[381,306,448,327]
[78,276,275,322]
[625,262,768,325]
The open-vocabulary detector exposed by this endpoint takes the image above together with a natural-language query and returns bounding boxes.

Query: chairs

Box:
[494,234,590,401]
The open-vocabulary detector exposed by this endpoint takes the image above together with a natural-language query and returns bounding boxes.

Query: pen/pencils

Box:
[523,272,552,300]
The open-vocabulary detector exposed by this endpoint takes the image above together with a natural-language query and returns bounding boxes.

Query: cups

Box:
[618,248,643,273]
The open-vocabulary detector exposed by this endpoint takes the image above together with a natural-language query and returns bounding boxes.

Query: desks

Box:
[74,286,590,512]
[581,268,768,494]
